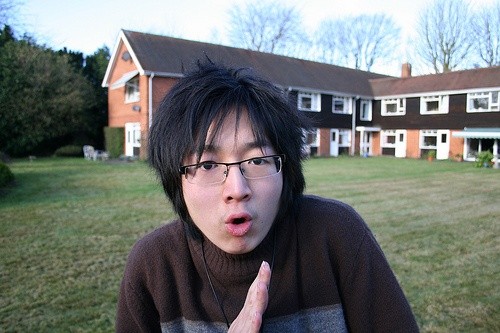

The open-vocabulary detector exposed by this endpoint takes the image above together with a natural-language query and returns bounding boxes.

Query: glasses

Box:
[179,154,285,185]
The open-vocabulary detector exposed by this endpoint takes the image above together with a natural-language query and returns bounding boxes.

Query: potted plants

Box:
[456,154,463,162]
[427,151,435,161]
[475,149,494,168]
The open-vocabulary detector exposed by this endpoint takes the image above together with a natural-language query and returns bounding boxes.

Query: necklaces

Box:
[201,232,279,330]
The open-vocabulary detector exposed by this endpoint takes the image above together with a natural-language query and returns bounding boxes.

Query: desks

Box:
[90,154,106,161]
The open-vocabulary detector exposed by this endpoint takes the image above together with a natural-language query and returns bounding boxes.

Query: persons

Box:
[114,50,420,333]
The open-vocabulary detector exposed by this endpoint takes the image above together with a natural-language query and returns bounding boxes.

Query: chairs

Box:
[94,150,108,161]
[83,145,95,160]
